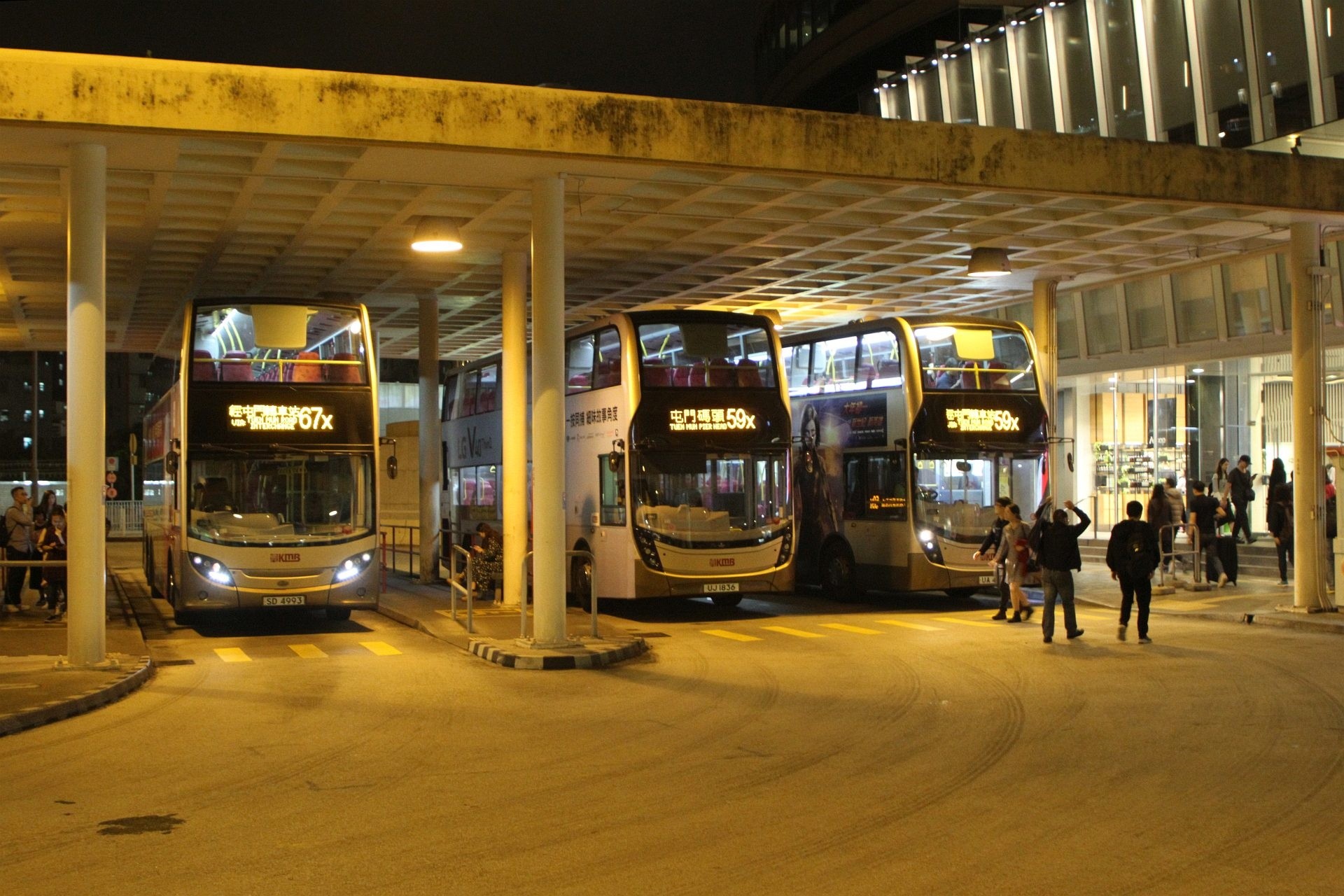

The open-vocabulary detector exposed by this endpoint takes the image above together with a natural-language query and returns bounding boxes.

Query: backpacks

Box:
[1127,530,1152,579]
[1274,501,1294,543]
[0,507,21,547]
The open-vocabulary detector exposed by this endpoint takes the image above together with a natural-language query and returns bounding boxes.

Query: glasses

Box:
[20,492,28,496]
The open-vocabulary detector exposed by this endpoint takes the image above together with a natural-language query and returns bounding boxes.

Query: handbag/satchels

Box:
[1028,502,1050,552]
[1207,483,1213,497]
[1026,558,1040,572]
[1246,488,1255,501]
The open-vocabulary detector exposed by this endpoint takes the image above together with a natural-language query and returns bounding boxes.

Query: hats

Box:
[1240,455,1252,464]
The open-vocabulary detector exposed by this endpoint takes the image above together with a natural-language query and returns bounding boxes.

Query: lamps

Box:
[411,216,462,252]
[967,247,1012,276]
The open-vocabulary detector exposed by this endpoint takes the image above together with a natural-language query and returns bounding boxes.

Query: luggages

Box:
[1205,518,1237,586]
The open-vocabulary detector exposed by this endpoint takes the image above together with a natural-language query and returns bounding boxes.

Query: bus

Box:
[727,313,1075,605]
[440,301,796,615]
[137,294,398,623]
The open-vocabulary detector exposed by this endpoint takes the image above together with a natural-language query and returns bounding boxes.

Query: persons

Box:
[30,505,52,610]
[958,468,981,490]
[791,402,840,558]
[447,522,502,601]
[1287,470,1293,485]
[1265,458,1286,539]
[1220,454,1258,545]
[1163,476,1188,574]
[2,487,34,611]
[973,497,1011,621]
[1105,500,1160,645]
[1324,467,1336,594]
[1034,496,1091,642]
[1212,458,1244,540]
[986,503,1034,623]
[1272,484,1295,586]
[1146,483,1173,587]
[37,506,67,623]
[935,357,962,389]
[37,489,63,524]
[1188,480,1228,588]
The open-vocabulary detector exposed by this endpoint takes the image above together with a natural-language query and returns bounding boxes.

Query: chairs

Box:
[655,471,779,521]
[447,357,765,420]
[802,357,1011,392]
[190,349,364,382]
[202,477,235,512]
[460,476,496,505]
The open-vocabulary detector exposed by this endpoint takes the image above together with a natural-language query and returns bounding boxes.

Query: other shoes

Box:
[1159,564,1168,573]
[461,589,477,601]
[1044,636,1052,642]
[1182,559,1188,572]
[1277,580,1289,586]
[2,596,66,622]
[1067,629,1084,639]
[1138,633,1152,644]
[1024,609,1033,620]
[1118,625,1126,641]
[1233,536,1245,544]
[1326,588,1334,593]
[1218,572,1228,588]
[1008,611,1021,622]
[455,572,460,584]
[1248,537,1257,544]
[992,611,1006,620]
